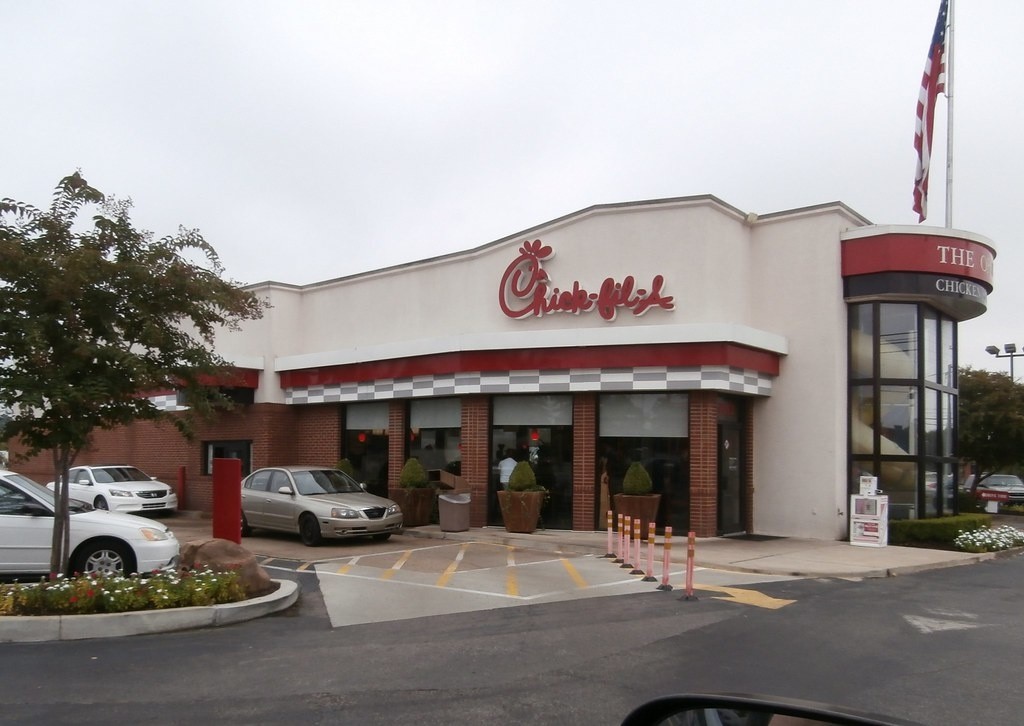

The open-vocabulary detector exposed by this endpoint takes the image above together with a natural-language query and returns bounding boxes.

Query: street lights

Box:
[986,343,1024,385]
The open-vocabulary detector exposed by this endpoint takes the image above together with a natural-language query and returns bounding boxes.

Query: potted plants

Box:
[613,460,662,540]
[388,457,434,527]
[496,461,552,533]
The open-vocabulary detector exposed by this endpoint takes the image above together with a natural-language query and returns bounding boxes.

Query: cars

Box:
[0,468,182,583]
[925,472,937,501]
[44,462,179,518]
[964,472,1024,506]
[240,465,405,547]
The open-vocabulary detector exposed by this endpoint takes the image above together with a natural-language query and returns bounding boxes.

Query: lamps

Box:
[530,428,539,440]
